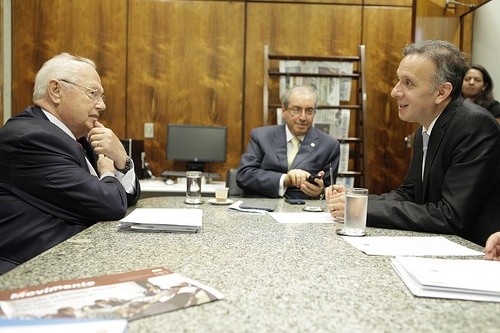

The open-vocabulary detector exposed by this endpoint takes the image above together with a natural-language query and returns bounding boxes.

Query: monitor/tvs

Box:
[165,124,227,172]
[118,139,144,179]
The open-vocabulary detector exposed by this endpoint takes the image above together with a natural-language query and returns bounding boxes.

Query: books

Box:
[228,200,278,214]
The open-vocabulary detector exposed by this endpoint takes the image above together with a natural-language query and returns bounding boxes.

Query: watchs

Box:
[114,157,133,174]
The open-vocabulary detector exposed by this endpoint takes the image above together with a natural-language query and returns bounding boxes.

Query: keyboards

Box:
[160,171,222,179]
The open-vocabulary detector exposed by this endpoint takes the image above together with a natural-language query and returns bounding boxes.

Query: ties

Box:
[288,138,300,169]
[76,141,89,160]
[422,133,429,183]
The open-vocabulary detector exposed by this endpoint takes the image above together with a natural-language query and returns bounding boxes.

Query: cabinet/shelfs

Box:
[264,45,365,190]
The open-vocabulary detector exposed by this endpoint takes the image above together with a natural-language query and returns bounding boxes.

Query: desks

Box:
[138,178,227,195]
[0,196,500,333]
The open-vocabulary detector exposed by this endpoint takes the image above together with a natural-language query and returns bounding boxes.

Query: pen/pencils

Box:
[330,163,333,194]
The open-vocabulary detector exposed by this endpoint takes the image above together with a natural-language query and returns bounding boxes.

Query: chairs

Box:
[227,169,247,196]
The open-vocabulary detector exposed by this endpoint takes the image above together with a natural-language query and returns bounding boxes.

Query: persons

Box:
[0,52,141,275]
[236,85,340,198]
[58,281,162,319]
[484,232,500,261]
[327,39,500,247]
[460,64,500,123]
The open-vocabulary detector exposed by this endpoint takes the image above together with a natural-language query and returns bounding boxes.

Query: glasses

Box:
[58,79,105,103]
[287,106,317,116]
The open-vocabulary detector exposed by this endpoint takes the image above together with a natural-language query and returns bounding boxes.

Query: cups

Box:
[343,187,369,234]
[185,171,202,203]
[215,188,229,202]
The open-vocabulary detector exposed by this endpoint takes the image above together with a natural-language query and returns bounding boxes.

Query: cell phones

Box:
[284,199,305,205]
[302,206,324,212]
[308,174,321,185]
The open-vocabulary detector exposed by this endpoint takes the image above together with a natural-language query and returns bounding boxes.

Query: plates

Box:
[208,199,233,204]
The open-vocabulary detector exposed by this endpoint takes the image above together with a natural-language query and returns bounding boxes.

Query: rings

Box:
[331,205,334,210]
